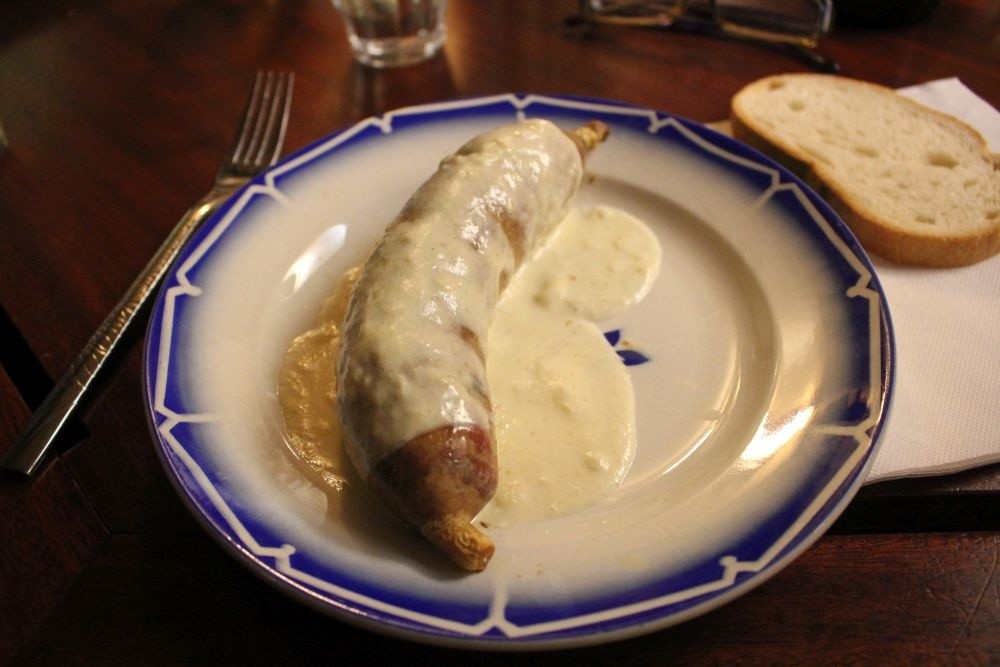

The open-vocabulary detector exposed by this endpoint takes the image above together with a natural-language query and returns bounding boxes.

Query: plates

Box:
[139,92,897,654]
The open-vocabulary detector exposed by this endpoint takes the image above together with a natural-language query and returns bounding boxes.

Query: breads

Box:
[730,73,1000,270]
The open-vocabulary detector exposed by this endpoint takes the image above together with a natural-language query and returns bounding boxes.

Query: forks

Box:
[6,67,297,474]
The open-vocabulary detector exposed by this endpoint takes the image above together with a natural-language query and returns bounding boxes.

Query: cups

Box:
[333,0,449,67]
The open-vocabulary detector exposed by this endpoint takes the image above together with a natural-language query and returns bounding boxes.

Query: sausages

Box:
[334,114,610,569]
